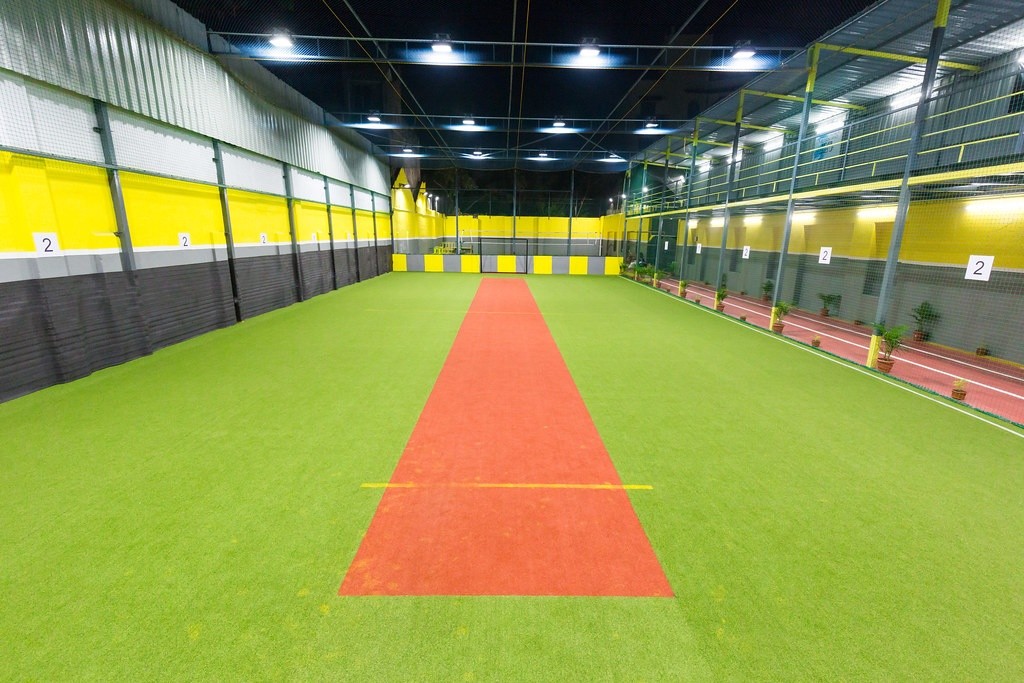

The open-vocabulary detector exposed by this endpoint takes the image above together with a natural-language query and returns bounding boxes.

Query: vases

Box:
[855,320,860,325]
[739,316,747,322]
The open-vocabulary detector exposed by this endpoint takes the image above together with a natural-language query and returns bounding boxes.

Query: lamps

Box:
[366,114,661,201]
[730,47,758,60]
[579,47,601,58]
[430,41,454,53]
[267,35,294,50]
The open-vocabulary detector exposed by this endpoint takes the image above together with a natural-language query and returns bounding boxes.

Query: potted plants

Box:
[761,280,773,301]
[772,307,784,336]
[812,336,821,348]
[907,302,942,343]
[871,322,911,373]
[705,271,746,312]
[952,379,967,401]
[620,246,700,305]
[976,344,990,356]
[817,293,841,316]
